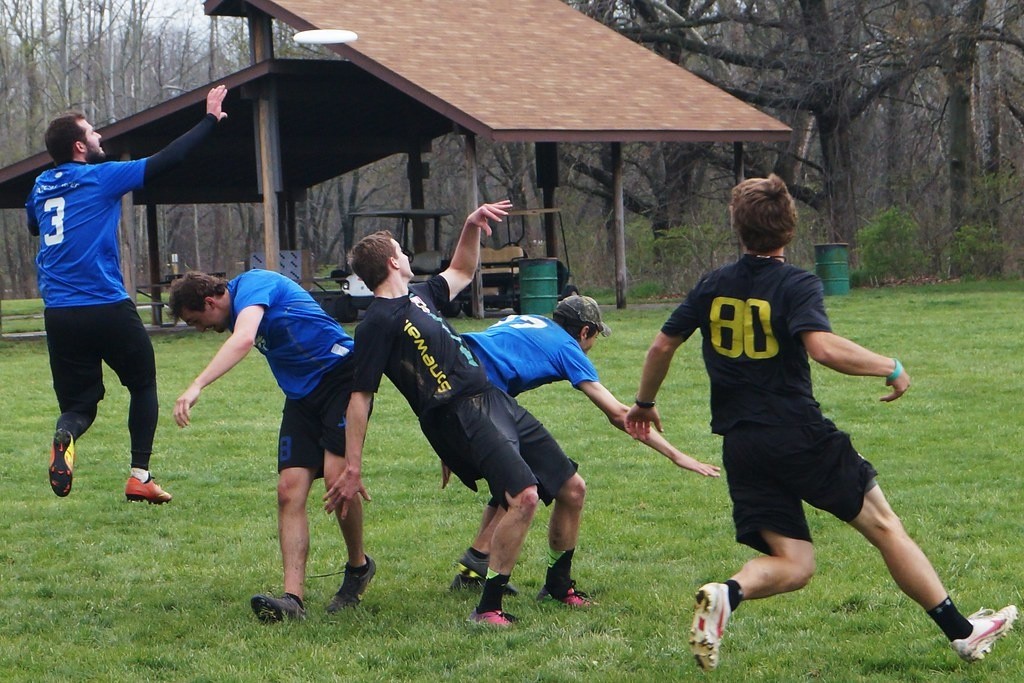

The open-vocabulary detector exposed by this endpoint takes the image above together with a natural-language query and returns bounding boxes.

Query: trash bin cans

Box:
[813,244,851,297]
[519,258,559,316]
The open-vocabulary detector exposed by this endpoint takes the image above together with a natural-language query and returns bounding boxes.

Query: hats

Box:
[554,295,613,339]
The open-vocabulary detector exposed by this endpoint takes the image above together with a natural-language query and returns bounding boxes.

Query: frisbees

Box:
[293,29,357,45]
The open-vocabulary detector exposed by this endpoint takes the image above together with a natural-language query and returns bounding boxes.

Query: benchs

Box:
[407,246,526,298]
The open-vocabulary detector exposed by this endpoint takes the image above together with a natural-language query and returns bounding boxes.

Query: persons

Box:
[167,267,376,623]
[445,294,722,597]
[624,170,1019,672]
[25,86,229,501]
[321,200,600,627]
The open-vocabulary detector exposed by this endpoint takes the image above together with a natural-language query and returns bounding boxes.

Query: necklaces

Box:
[755,254,787,263]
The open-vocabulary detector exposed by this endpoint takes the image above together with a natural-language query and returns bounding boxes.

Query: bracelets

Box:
[887,358,903,381]
[635,398,656,408]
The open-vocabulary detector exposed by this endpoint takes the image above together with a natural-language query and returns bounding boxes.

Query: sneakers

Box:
[950,604,1018,663]
[124,473,171,505]
[325,553,375,614]
[690,581,730,670]
[451,547,518,596]
[250,591,307,625]
[536,586,599,610]
[48,429,75,499]
[468,605,518,629]
